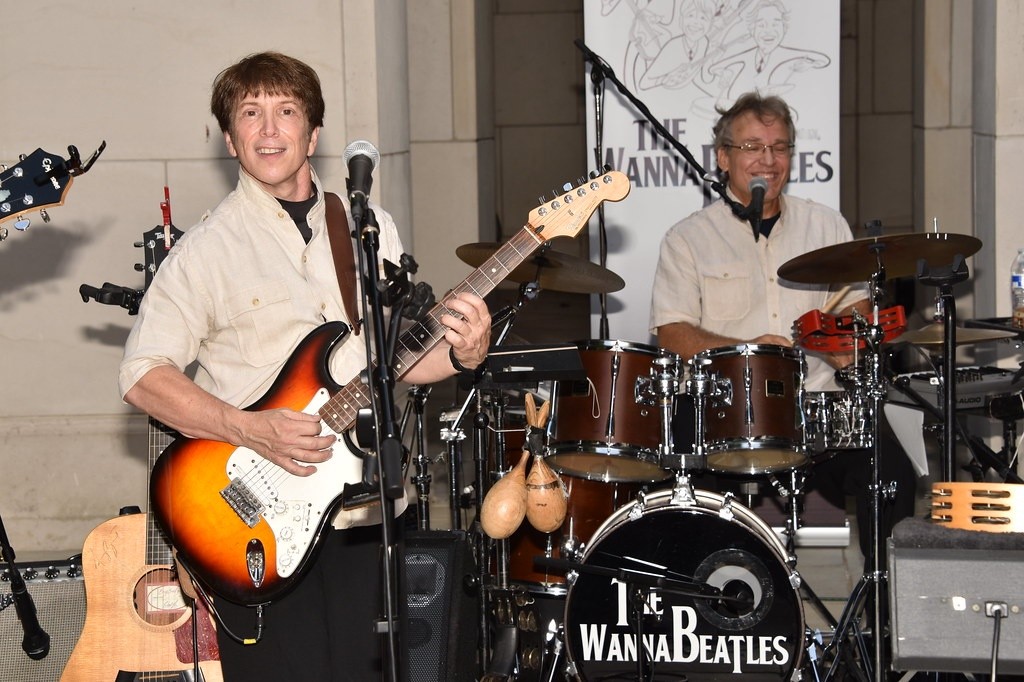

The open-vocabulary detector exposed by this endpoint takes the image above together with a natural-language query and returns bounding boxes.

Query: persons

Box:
[649,92,920,682]
[118,52,492,682]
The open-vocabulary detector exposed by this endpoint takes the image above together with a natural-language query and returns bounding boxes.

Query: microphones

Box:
[723,587,755,606]
[342,139,380,220]
[9,568,50,660]
[748,177,768,240]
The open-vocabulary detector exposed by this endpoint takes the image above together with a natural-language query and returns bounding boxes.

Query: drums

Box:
[540,337,678,486]
[488,464,634,597]
[691,340,812,477]
[562,487,806,682]
[801,388,875,452]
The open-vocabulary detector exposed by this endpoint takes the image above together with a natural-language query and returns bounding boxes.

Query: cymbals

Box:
[885,321,1019,345]
[925,480,1023,535]
[457,241,628,297]
[776,231,983,287]
[789,304,908,355]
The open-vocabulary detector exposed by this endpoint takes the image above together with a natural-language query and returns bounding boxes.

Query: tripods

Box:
[787,248,978,682]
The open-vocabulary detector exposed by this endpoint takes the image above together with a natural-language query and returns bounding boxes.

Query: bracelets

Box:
[450,346,472,372]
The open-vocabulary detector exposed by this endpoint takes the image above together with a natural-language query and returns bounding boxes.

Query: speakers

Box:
[401,530,481,682]
[0,549,88,682]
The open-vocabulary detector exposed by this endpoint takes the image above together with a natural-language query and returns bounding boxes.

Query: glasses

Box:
[726,142,796,156]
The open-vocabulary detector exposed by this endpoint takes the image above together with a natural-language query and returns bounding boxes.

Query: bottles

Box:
[1010,247,1024,329]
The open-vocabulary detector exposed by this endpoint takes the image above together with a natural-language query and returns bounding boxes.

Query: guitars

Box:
[1,136,110,244]
[148,164,634,613]
[57,185,227,682]
